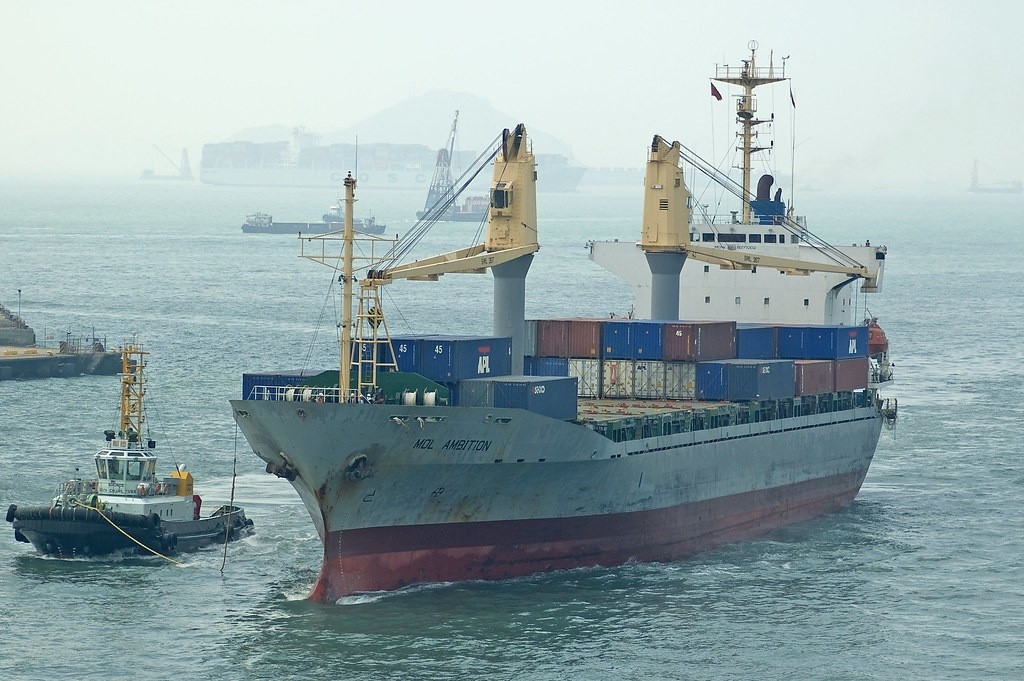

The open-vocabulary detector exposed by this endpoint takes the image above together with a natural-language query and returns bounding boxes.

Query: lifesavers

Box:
[67,497,78,505]
[162,532,178,550]
[15,527,31,542]
[147,513,160,530]
[89,495,98,507]
[6,503,18,521]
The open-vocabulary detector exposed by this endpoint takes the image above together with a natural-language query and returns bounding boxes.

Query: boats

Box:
[5,339,255,563]
[239,201,388,237]
[228,33,897,599]
[416,108,489,224]
